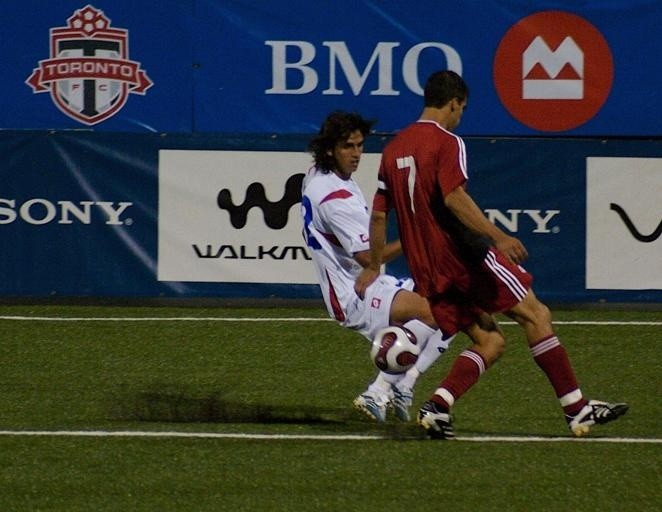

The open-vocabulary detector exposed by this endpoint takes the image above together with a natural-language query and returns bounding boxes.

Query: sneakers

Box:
[389,385,414,423]
[416,400,457,440]
[562,399,629,438]
[351,393,387,423]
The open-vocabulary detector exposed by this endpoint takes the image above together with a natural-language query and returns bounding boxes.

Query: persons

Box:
[301,112,457,420]
[354,71,629,441]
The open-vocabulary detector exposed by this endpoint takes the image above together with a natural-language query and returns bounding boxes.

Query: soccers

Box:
[370,326,421,375]
